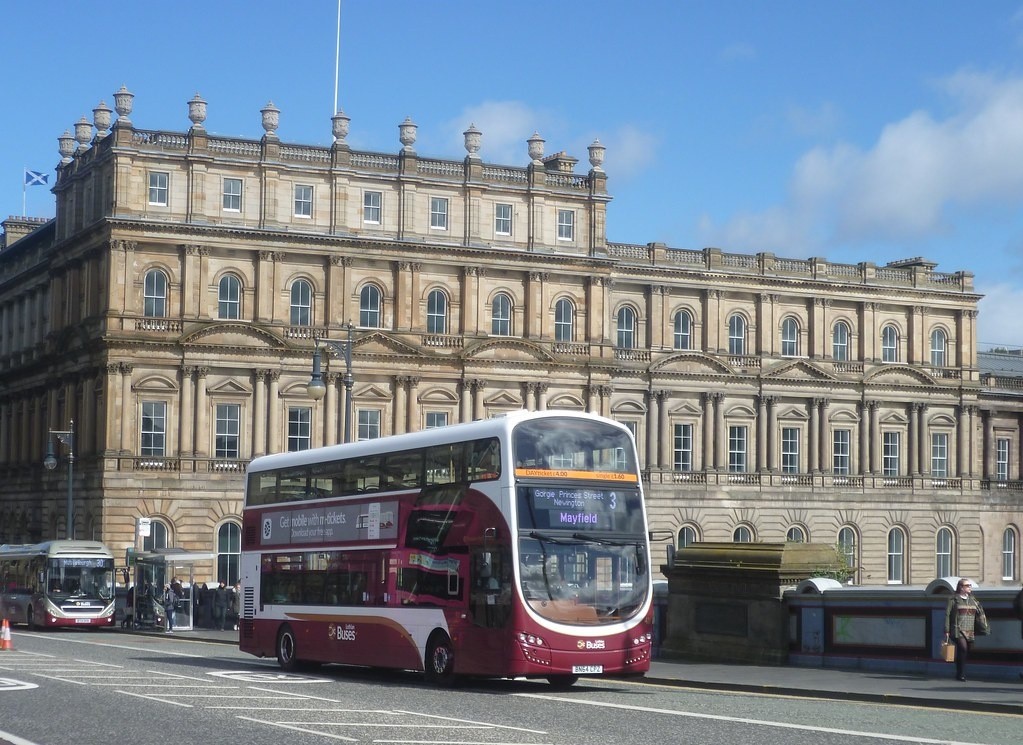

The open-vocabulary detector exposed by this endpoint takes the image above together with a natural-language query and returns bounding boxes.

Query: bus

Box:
[238,410,652,685]
[0,539,116,629]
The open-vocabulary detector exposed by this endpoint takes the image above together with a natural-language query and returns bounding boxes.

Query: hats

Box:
[164,582,172,590]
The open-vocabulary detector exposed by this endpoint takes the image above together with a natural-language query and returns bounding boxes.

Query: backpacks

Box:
[171,591,181,608]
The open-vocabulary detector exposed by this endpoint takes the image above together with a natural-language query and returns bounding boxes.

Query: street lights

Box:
[308,318,355,444]
[44,418,73,539]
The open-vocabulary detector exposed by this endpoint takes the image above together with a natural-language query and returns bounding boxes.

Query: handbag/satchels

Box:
[941,634,954,662]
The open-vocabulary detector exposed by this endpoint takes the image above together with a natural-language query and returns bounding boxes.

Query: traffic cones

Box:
[0,619,17,652]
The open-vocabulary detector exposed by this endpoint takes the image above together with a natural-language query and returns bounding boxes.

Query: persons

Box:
[232,579,241,631]
[121,577,214,634]
[213,579,230,632]
[1013,589,1023,679]
[52,579,62,592]
[943,578,993,682]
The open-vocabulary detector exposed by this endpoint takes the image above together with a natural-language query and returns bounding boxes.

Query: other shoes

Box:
[956,674,967,681]
[166,630,173,633]
[234,625,238,630]
[121,621,124,628]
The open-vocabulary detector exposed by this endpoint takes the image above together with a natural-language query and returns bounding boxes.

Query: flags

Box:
[26,171,49,186]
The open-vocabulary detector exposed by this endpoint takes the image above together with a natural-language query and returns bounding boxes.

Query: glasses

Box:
[963,584,972,587]
